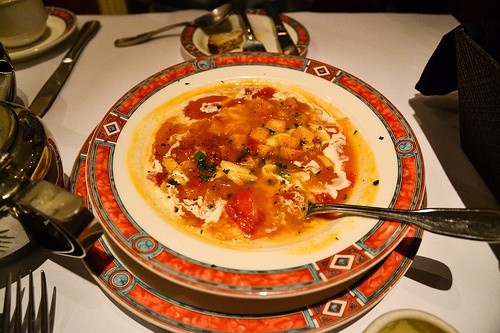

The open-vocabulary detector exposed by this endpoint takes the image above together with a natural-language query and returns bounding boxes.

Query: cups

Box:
[0,0,49,46]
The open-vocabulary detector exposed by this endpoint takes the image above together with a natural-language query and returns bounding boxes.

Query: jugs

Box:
[0,98,106,270]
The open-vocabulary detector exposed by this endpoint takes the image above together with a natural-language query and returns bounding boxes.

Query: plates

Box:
[70,115,428,333]
[179,15,310,59]
[84,53,425,301]
[0,6,77,60]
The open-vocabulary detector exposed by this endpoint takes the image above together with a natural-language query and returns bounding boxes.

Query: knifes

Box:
[265,2,301,56]
[27,20,102,118]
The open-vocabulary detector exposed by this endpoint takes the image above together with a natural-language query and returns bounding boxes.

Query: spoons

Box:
[304,201,500,243]
[113,0,236,47]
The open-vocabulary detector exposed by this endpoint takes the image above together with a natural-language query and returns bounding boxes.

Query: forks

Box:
[237,0,267,54]
[0,270,57,333]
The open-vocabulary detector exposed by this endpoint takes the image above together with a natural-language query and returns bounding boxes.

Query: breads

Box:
[207,29,244,54]
